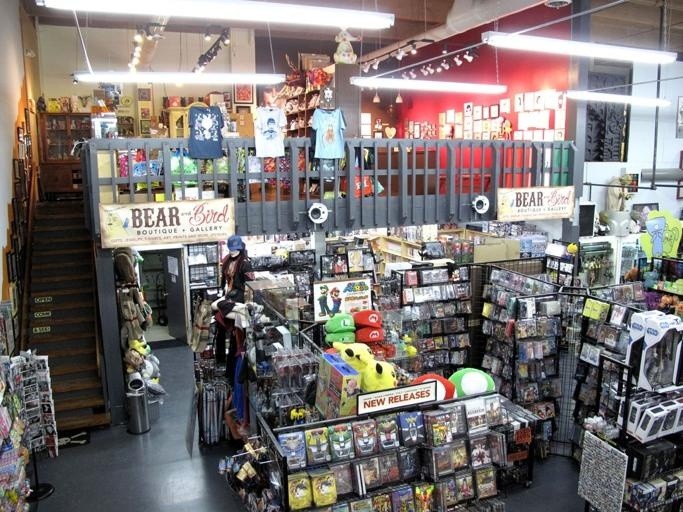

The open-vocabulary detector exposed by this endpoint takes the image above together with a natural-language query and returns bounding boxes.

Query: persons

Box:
[628,210,641,234]
[211,235,256,310]
[606,175,632,211]
[598,212,610,236]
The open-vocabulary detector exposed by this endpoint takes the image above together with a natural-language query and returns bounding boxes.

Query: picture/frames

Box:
[632,202,658,228]
[235,106,251,114]
[6,107,33,284]
[373,89,567,139]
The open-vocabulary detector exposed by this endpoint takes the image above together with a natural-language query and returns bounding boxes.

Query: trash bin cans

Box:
[125,378,151,435]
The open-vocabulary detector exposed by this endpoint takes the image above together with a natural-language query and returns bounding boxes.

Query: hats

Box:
[325,310,383,346]
[227,235,243,250]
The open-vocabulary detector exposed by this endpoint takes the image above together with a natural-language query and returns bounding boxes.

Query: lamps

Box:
[566,88,671,108]
[119,23,233,72]
[354,36,479,85]
[479,30,677,67]
[69,67,288,93]
[348,75,511,98]
[38,0,398,34]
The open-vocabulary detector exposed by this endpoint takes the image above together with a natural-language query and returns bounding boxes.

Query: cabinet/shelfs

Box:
[186,228,683,511]
[1,381,40,512]
[262,73,339,200]
[36,112,93,201]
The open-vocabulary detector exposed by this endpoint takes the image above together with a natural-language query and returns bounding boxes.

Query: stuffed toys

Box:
[113,248,167,395]
[333,342,397,393]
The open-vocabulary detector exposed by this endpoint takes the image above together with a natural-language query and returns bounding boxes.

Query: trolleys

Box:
[156,271,167,326]
